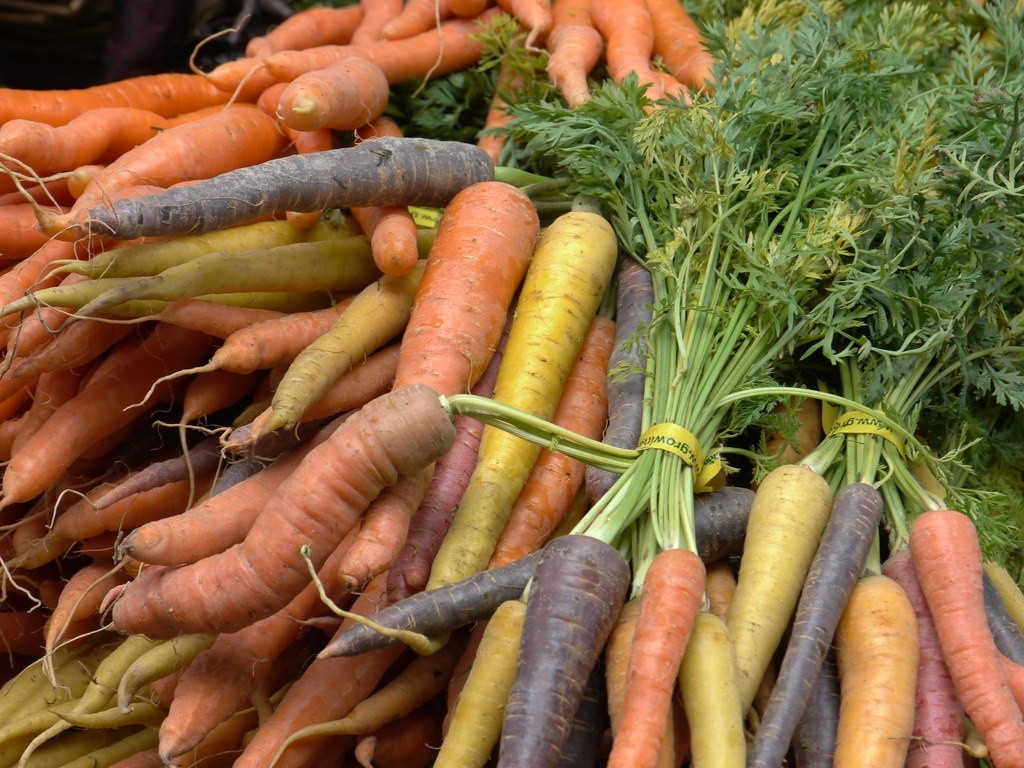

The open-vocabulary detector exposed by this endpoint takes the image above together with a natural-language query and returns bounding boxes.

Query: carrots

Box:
[0,0,1024,768]
